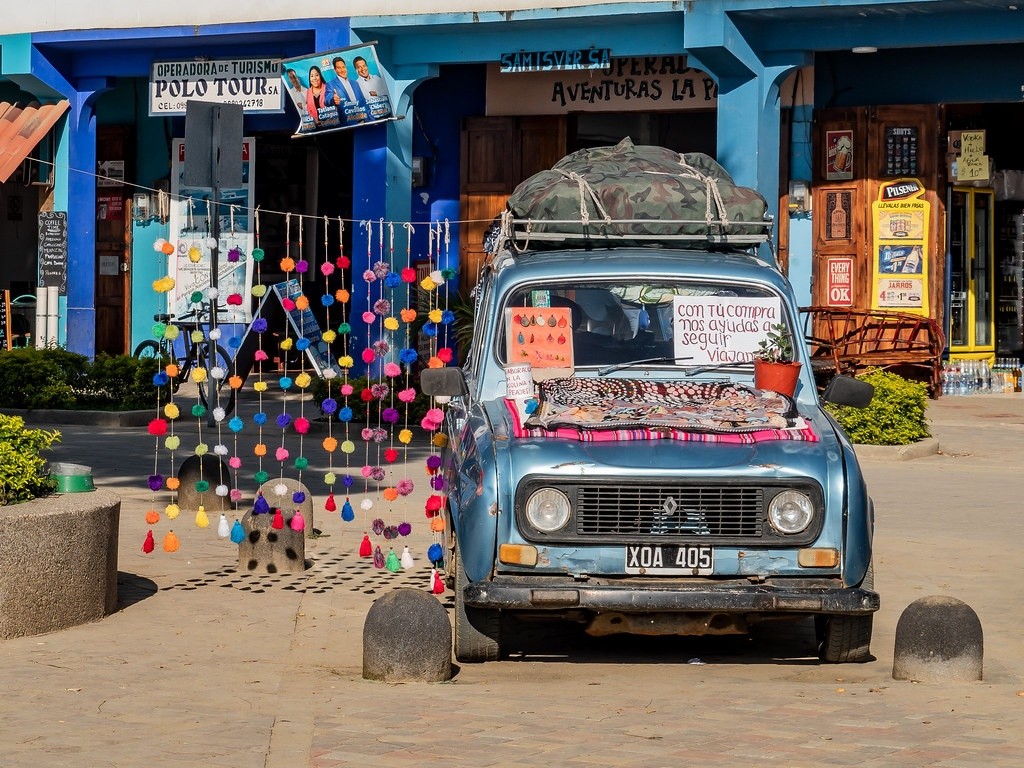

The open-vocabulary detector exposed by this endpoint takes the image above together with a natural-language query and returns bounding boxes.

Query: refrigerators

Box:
[994,198,1024,365]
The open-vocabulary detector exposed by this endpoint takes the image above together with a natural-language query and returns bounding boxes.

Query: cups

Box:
[833,151,852,172]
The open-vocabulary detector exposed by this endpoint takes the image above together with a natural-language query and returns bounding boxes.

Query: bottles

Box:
[998,304,1018,323]
[941,357,1022,396]
[831,193,846,237]
[1000,208,1017,240]
[1001,274,1017,295]
[1004,247,1015,266]
[882,246,894,272]
[902,249,919,273]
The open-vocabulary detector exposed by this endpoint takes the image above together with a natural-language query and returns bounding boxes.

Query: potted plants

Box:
[751,322,802,398]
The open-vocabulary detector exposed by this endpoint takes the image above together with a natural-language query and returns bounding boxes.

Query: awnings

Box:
[0,98,71,186]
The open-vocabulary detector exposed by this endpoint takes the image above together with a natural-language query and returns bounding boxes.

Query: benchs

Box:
[798,307,948,401]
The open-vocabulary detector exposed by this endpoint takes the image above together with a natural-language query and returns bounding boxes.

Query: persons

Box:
[324,57,376,125]
[287,69,316,131]
[353,57,392,120]
[305,66,327,129]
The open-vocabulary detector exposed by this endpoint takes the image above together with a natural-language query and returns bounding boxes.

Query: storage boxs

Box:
[947,129,993,186]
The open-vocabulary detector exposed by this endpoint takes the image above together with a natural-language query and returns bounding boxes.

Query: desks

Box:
[9,302,37,346]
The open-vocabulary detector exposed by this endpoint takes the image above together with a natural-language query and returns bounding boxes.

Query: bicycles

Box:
[132,303,235,417]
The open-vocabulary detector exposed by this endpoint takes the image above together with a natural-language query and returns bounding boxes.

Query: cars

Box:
[422,227,885,665]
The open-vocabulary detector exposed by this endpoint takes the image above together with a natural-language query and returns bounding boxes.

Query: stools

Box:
[10,333,30,349]
